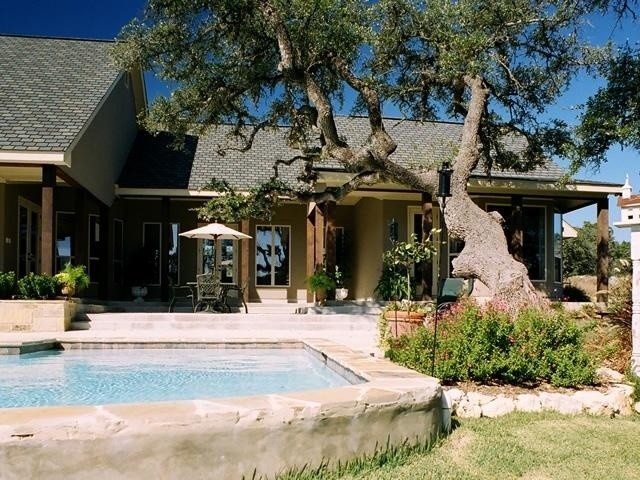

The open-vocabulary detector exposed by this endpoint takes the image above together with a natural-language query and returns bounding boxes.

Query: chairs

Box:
[167,270,251,313]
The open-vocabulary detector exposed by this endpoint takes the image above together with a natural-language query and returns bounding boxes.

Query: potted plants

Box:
[373,227,446,338]
[53,262,89,294]
[306,265,348,307]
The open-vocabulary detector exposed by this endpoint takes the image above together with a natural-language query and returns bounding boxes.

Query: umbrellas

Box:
[177,220,253,276]
